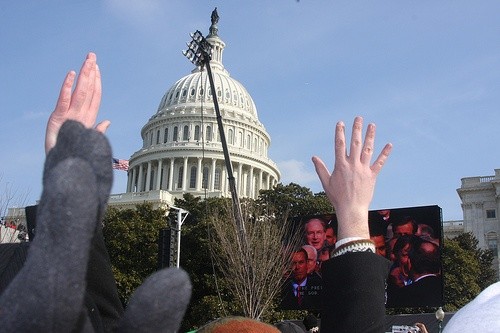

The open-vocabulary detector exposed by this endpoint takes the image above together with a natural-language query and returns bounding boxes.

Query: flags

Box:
[111,156,130,170]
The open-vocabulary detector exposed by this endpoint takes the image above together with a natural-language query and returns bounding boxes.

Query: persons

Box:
[0,50,191,333]
[274,208,444,312]
[414,323,429,333]
[198,114,394,333]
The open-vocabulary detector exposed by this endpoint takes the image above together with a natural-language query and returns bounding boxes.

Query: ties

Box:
[296,286,302,303]
